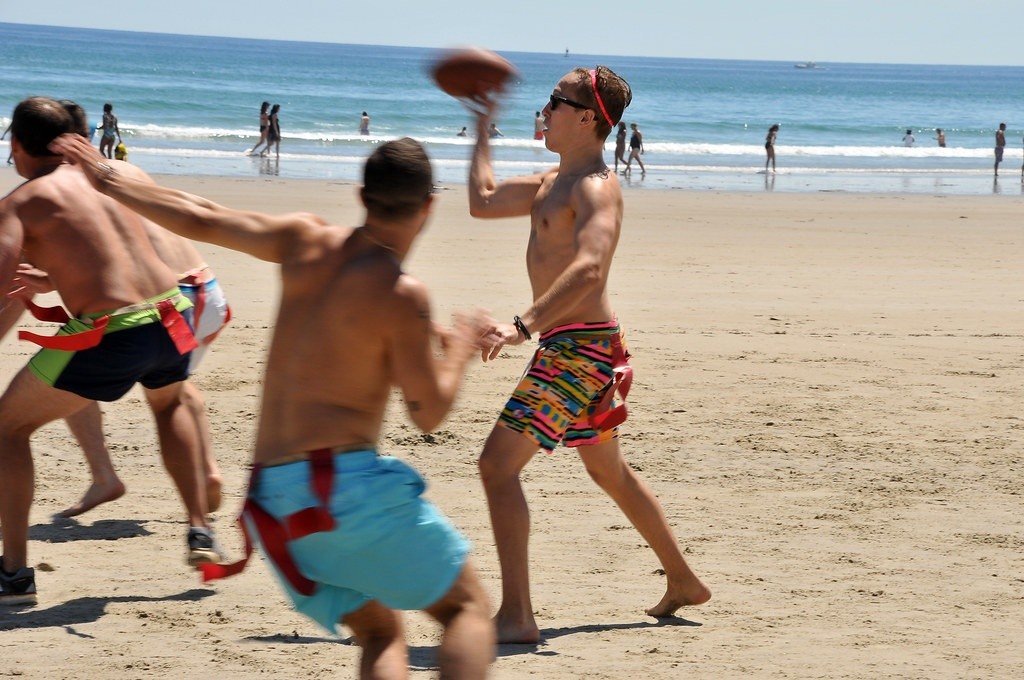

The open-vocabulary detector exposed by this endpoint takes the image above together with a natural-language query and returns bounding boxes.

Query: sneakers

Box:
[187,527,222,565]
[0,556,38,605]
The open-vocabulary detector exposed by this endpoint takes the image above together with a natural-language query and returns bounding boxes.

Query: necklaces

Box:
[362,232,406,254]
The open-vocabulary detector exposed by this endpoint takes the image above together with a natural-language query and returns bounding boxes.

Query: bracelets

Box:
[513,316,532,340]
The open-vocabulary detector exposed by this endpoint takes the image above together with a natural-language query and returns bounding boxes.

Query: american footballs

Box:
[433,49,516,98]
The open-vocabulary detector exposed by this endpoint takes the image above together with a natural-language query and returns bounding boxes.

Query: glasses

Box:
[549,94,599,121]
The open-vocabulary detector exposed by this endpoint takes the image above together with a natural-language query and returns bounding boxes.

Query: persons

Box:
[96,103,121,159]
[361,111,369,129]
[0,95,232,604]
[901,130,915,147]
[613,122,645,174]
[765,124,779,171]
[463,67,711,642]
[251,101,280,157]
[534,111,544,139]
[462,127,466,135]
[48,132,498,679]
[933,129,945,147]
[994,123,1006,176]
[488,123,502,136]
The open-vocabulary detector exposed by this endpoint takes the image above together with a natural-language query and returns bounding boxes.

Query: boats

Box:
[795,61,826,69]
